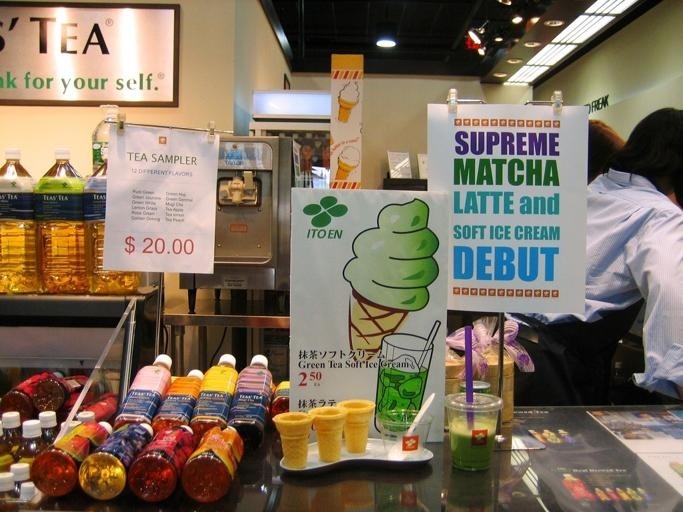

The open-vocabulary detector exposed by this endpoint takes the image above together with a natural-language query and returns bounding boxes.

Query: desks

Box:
[161,295,290,373]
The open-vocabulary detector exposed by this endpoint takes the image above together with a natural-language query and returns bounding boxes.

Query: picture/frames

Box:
[0,0,180,108]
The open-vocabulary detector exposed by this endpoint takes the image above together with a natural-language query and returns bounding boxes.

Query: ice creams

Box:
[338,82,359,123]
[334,147,360,180]
[343,198,439,361]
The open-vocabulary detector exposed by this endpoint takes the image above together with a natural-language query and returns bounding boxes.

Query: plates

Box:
[278,435,434,471]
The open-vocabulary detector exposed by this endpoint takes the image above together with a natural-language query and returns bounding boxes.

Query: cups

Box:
[375,409,434,461]
[444,392,505,472]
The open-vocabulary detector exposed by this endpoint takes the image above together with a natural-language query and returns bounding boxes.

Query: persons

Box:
[516,108,683,406]
[586,117,628,187]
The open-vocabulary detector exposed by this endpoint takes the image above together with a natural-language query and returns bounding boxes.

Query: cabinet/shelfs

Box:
[0,406,683,512]
[0,285,164,400]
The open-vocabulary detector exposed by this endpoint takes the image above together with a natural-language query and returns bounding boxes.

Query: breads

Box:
[445,347,467,424]
[467,352,513,424]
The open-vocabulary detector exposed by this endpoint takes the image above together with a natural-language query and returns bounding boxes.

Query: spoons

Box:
[388,392,436,460]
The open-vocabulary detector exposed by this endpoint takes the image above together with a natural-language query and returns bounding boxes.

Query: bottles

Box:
[190,354,236,432]
[271,380,290,415]
[93,105,125,171]
[113,354,173,428]
[32,375,103,415]
[636,487,652,505]
[151,370,204,430]
[79,410,96,423]
[457,380,491,395]
[19,482,36,511]
[6,411,21,450]
[78,395,122,425]
[594,488,611,504]
[29,422,113,496]
[563,473,597,508]
[607,488,620,502]
[78,423,153,500]
[628,487,642,506]
[63,421,80,433]
[127,425,195,502]
[182,425,244,504]
[530,429,576,449]
[38,411,58,446]
[34,151,84,293]
[1,148,36,293]
[6,473,20,510]
[82,149,139,294]
[616,488,632,507]
[14,420,47,463]
[227,354,272,449]
[10,463,30,481]
[0,372,63,419]
